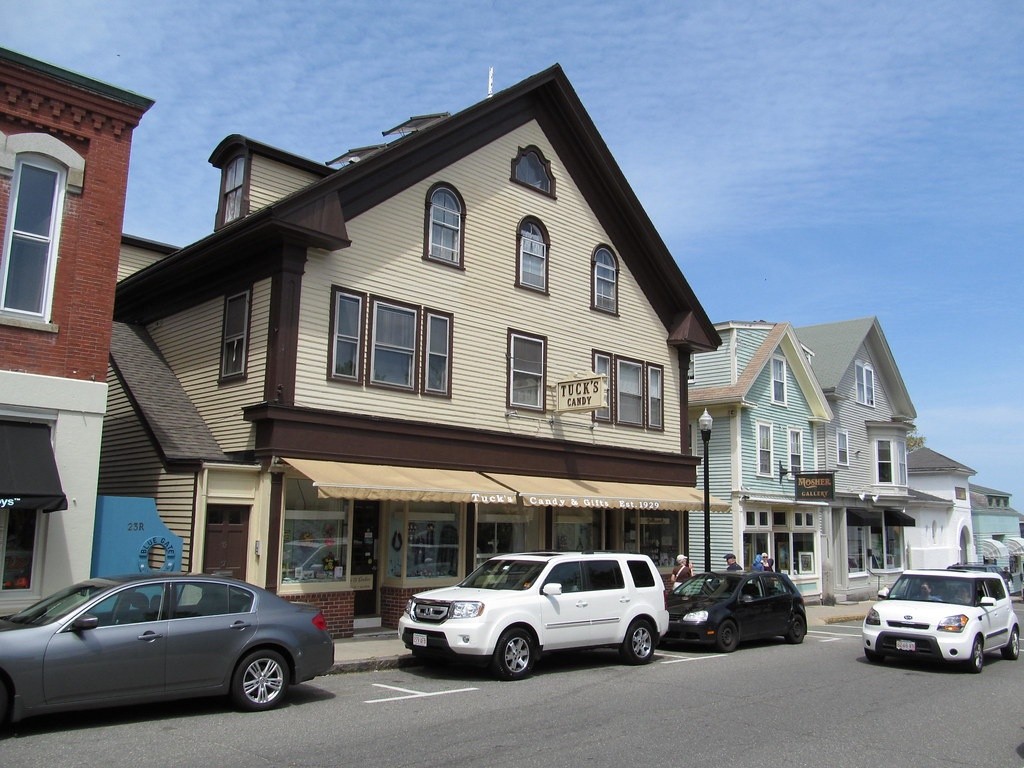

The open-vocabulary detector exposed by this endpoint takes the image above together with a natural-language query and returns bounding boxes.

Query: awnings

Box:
[846,509,915,527]
[983,538,1024,559]
[281,459,733,515]
[0,419,70,512]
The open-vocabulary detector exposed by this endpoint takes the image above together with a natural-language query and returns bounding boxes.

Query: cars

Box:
[662,568,807,654]
[0,571,336,728]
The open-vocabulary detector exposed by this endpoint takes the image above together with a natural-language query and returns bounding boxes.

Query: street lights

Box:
[698,407,713,572]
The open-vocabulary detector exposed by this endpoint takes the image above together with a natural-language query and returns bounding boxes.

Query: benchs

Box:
[192,590,250,615]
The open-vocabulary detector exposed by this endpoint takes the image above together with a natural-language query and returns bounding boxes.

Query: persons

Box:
[951,585,971,604]
[913,582,933,600]
[752,552,774,572]
[723,554,742,571]
[1004,568,1014,593]
[671,555,694,593]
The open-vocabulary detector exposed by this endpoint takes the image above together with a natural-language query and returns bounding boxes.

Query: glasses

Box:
[763,556,767,559]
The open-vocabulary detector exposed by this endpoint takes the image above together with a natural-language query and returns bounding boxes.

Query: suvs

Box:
[283,535,378,582]
[396,546,670,681]
[860,566,1022,675]
[945,562,1012,598]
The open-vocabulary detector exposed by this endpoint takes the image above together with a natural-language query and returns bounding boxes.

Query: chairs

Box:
[126,594,161,623]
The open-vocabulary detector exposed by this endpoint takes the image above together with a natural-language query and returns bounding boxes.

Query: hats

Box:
[723,554,736,559]
[676,555,687,564]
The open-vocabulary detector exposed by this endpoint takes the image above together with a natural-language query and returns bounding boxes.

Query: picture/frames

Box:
[744,543,752,572]
[798,552,815,574]
